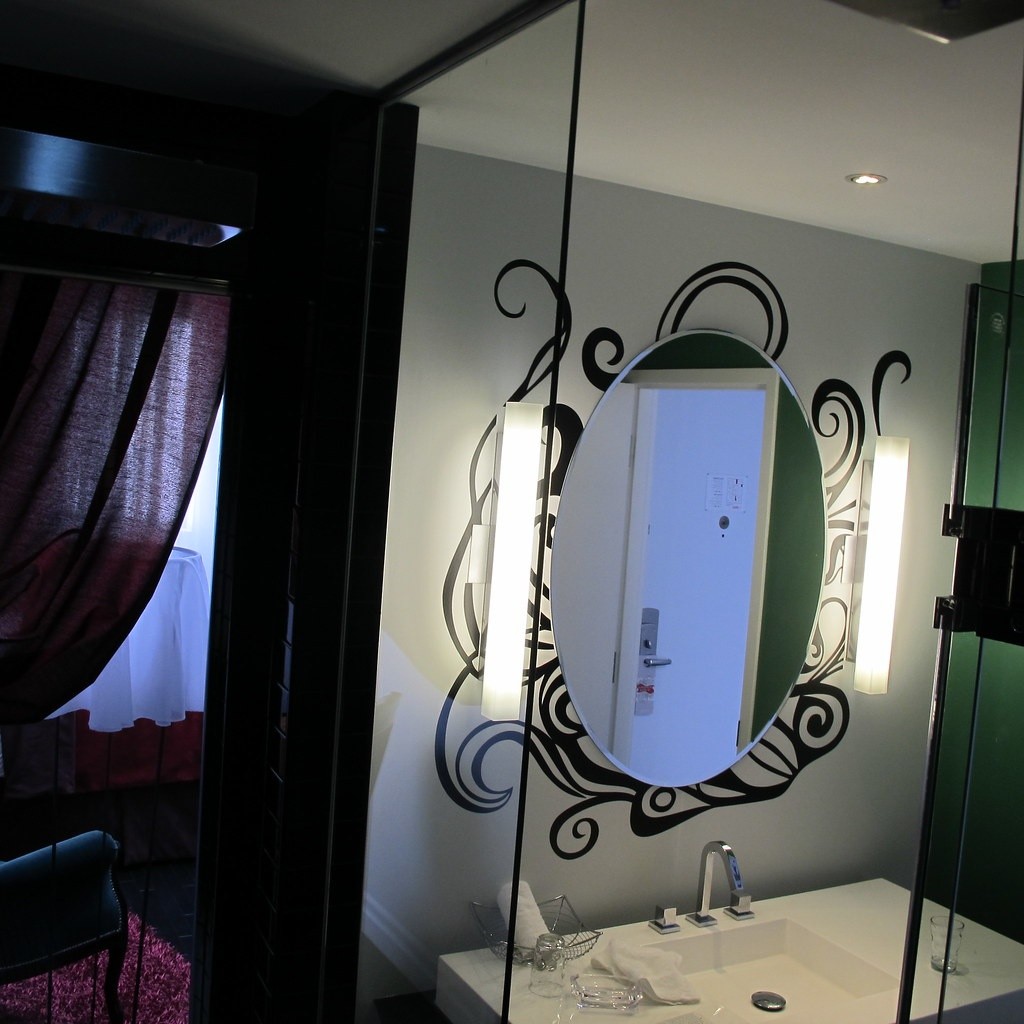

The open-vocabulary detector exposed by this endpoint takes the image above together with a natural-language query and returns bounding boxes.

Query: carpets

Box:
[0,903,189,1024]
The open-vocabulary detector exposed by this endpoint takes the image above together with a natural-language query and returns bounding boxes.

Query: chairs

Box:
[2,826,130,1024]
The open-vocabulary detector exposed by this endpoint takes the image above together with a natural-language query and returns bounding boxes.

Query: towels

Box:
[498,876,556,959]
[589,937,703,1008]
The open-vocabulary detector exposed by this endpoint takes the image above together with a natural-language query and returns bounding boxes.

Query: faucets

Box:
[685,839,753,929]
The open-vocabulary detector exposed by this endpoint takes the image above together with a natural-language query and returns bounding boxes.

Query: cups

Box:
[930,915,964,973]
[528,932,565,999]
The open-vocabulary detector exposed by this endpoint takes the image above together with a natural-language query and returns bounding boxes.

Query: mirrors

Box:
[547,326,827,790]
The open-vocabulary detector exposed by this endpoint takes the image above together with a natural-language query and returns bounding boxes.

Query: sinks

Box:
[638,914,902,1024]
